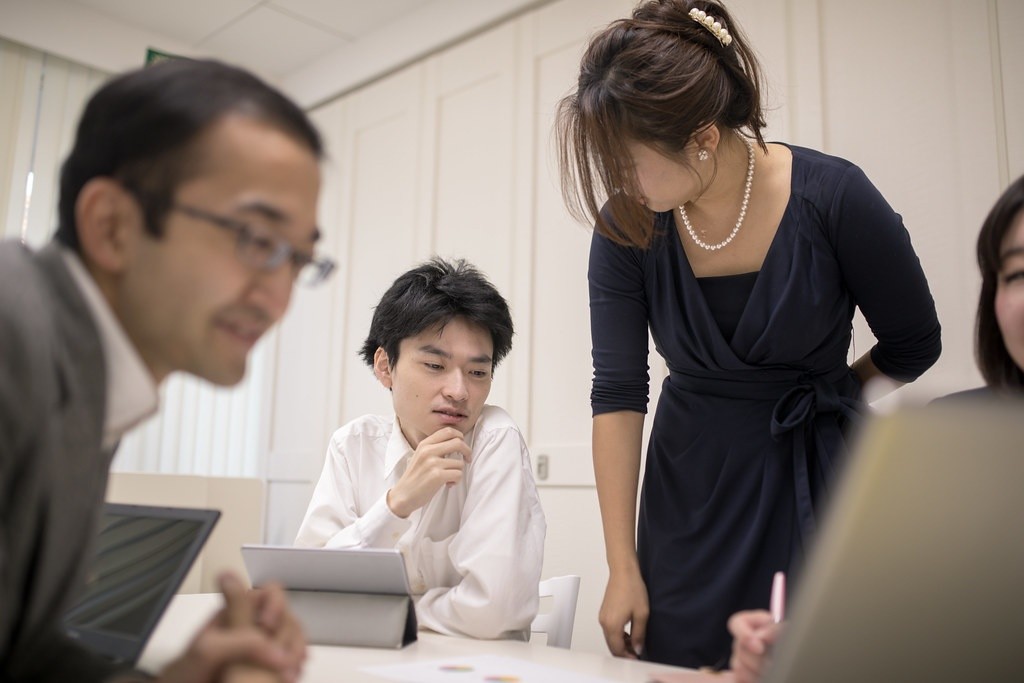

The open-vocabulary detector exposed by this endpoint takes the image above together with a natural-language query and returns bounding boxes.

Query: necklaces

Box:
[679,129,756,250]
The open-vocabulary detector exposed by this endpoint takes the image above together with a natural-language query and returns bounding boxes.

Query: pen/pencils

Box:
[770,571,785,625]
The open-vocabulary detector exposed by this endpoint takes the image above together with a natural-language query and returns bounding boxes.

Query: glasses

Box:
[128,194,336,288]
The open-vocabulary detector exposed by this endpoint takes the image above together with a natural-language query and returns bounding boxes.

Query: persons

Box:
[555,0,940,673]
[293,261,547,641]
[0,61,322,683]
[729,171,1024,683]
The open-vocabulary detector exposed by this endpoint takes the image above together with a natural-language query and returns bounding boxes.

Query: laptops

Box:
[65,502,221,670]
[762,395,1023,683]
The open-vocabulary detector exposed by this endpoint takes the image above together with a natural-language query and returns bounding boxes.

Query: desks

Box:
[136,594,696,683]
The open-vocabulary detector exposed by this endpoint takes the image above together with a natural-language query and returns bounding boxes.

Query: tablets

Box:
[243,545,418,640]
